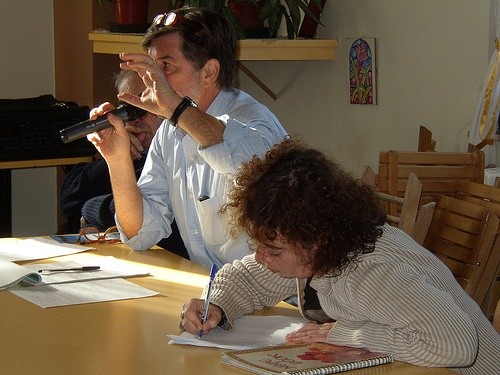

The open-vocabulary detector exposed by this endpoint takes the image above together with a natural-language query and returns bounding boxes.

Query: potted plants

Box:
[167,0,326,39]
[96,0,148,25]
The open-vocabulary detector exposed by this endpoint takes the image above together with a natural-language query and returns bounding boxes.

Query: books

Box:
[222,340,393,374]
[0,261,151,289]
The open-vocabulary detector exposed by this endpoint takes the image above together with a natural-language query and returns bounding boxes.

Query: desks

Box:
[0,233,460,375]
[0,155,95,238]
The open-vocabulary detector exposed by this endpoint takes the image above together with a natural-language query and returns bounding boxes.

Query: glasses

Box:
[76,226,122,245]
[153,12,201,29]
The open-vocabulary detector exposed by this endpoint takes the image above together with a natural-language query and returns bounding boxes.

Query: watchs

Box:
[168,96,198,126]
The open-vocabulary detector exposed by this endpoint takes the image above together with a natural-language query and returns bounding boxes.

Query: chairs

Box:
[373,126,500,326]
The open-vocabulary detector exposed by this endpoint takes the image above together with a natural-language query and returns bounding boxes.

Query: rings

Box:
[181,311,185,317]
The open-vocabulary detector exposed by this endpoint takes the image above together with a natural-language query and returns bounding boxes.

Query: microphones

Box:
[59,103,147,144]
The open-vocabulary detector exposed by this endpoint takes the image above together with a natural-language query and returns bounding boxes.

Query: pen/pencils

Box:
[198,264,217,340]
[38,266,100,273]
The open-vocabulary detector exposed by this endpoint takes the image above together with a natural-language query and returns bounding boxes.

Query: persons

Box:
[81,66,190,261]
[180,139,500,374]
[87,8,298,306]
